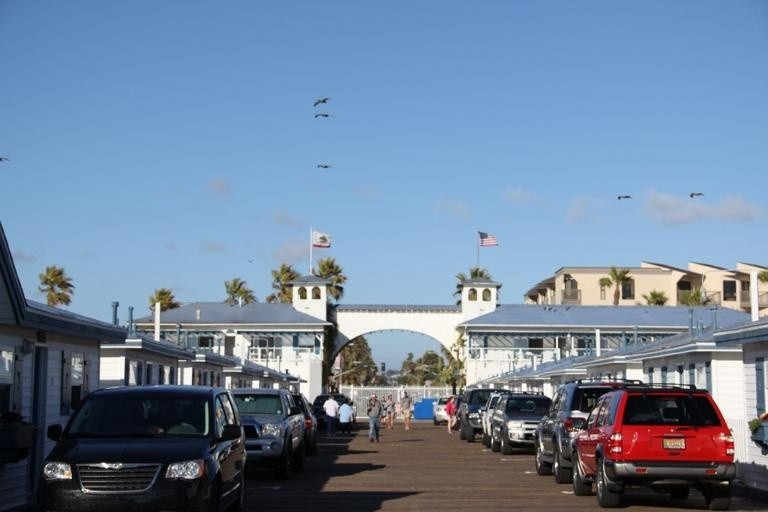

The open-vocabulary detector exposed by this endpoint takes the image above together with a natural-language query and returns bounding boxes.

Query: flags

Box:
[479,232,499,246]
[312,230,330,248]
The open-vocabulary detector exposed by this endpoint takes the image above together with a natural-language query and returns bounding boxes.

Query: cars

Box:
[312,391,357,434]
[432,387,554,457]
[285,389,320,459]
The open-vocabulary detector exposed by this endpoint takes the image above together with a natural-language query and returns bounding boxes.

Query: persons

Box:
[399,391,412,431]
[323,395,340,435]
[447,397,457,434]
[367,392,382,443]
[380,396,386,423]
[338,400,357,435]
[383,395,396,431]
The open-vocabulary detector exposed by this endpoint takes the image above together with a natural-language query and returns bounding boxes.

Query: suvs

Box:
[225,387,304,478]
[570,381,738,507]
[35,384,248,512]
[531,377,646,488]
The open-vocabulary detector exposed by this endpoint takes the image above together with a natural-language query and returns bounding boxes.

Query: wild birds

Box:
[0,157,10,161]
[314,113,334,119]
[618,195,631,200]
[690,192,704,198]
[314,97,332,107]
[316,163,333,169]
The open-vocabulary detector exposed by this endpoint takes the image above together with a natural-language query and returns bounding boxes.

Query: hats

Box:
[370,394,378,399]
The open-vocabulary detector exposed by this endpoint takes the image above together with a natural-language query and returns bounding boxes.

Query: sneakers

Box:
[369,438,380,443]
